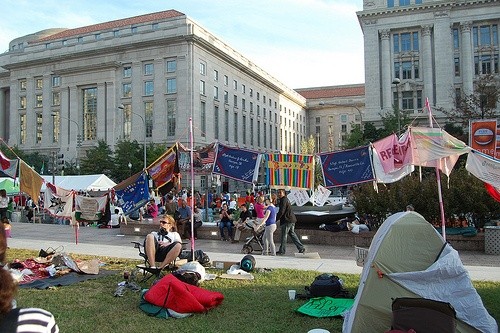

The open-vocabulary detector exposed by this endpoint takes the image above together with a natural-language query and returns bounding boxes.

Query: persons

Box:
[111,209,122,228]
[137,186,203,240]
[276,189,305,255]
[207,189,277,244]
[432,216,472,227]
[0,188,61,333]
[407,204,414,211]
[144,214,183,269]
[257,199,276,256]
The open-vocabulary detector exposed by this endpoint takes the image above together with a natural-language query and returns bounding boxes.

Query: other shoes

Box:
[231,240,239,244]
[276,252,285,256]
[238,226,245,230]
[299,248,305,253]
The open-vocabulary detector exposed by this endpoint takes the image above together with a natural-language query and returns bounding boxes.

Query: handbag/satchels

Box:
[296,273,354,299]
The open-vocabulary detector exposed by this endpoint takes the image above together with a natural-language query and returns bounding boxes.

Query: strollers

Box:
[240,219,269,255]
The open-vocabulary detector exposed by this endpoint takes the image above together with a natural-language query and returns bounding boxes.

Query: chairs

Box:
[132,237,188,282]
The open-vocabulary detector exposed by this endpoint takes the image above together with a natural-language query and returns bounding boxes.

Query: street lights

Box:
[318,101,366,142]
[50,113,81,171]
[118,105,148,169]
[391,78,402,137]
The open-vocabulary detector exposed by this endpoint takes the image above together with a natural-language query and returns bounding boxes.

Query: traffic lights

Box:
[55,152,65,172]
[49,152,55,171]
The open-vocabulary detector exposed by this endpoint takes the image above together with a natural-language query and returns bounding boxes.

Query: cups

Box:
[216,262,224,268]
[288,290,296,300]
[124,271,129,280]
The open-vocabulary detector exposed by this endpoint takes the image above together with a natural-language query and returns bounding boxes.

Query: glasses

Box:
[159,221,171,225]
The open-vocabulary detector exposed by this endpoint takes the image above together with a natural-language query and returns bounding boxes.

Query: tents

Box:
[342,212,498,333]
[0,174,118,217]
